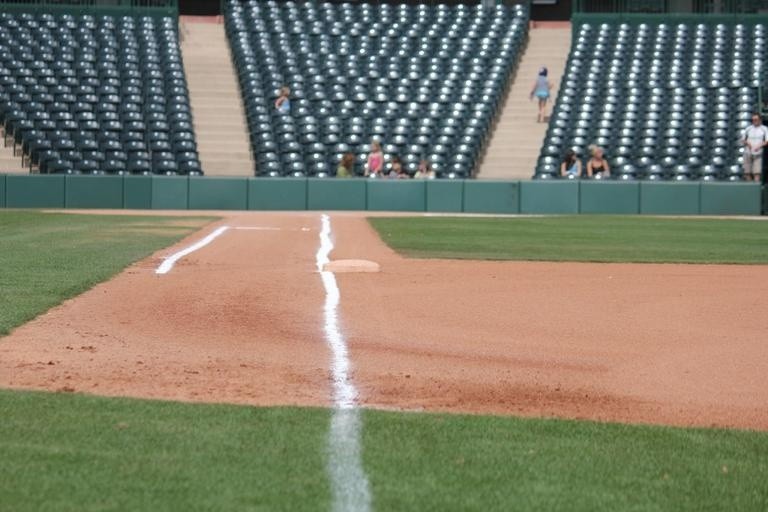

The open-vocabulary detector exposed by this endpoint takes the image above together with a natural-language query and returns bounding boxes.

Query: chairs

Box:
[0,12,206,178]
[533,21,768,184]
[223,2,529,178]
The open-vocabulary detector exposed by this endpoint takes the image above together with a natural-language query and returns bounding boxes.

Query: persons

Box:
[272,86,290,113]
[559,148,582,177]
[741,112,768,183]
[363,138,385,178]
[336,154,356,178]
[389,156,411,178]
[586,146,610,179]
[413,161,434,179]
[526,67,555,122]
